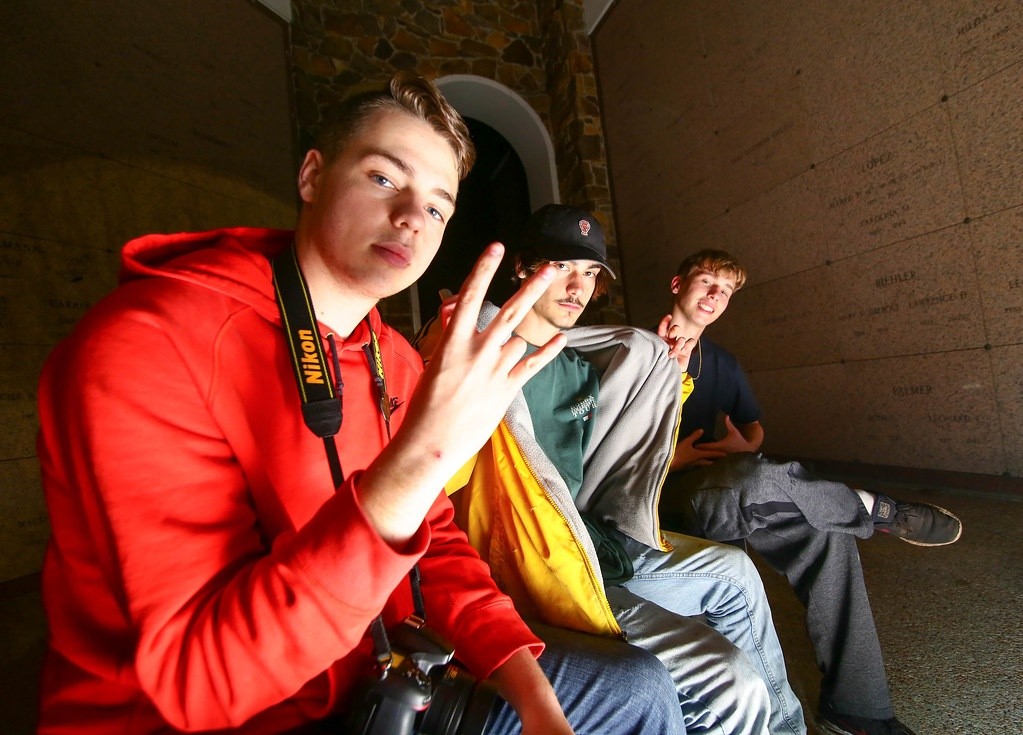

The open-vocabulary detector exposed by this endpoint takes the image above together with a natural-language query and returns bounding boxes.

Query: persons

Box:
[408,203,808,735]
[648,248,963,735]
[35,69,687,735]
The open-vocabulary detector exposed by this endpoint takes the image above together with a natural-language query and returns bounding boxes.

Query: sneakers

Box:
[817,710,916,735]
[864,490,963,546]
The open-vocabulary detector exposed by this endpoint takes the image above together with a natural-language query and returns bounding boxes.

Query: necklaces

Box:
[693,339,702,380]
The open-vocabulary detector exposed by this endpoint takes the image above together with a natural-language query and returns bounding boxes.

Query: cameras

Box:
[354,614,458,735]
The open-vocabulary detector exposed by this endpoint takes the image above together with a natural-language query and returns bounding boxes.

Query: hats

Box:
[519,203,616,281]
